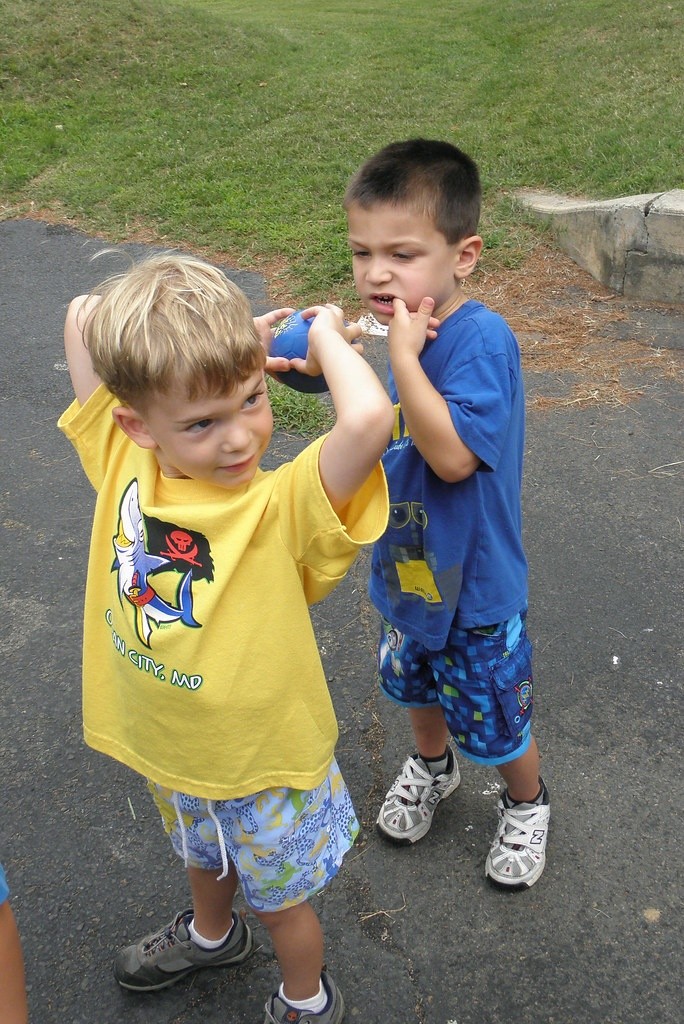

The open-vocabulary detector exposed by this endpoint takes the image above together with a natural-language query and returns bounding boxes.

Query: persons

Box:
[58,253,395,1024]
[342,138,551,891]
[0,862,29,1024]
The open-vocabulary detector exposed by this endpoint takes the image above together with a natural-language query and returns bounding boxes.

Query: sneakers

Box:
[484,775,551,891]
[111,909,257,991]
[375,743,461,845]
[263,971,346,1024]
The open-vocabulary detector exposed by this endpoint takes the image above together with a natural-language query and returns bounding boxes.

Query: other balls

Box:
[268,309,356,394]
